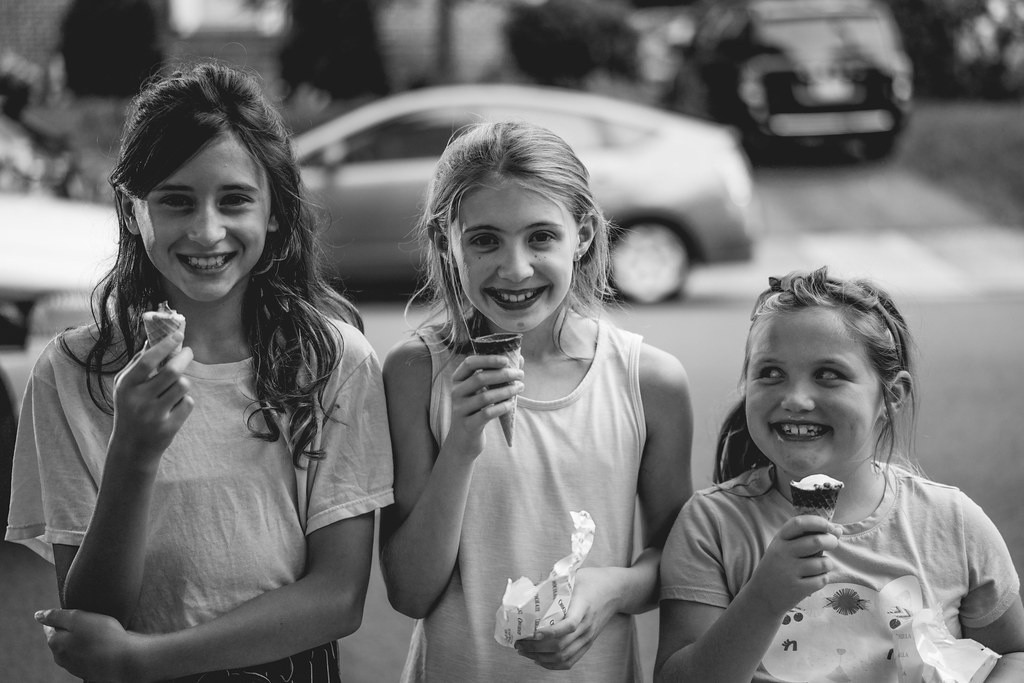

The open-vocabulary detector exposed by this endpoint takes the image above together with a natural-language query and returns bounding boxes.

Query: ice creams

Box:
[141,301,187,374]
[790,473,844,544]
[472,331,523,448]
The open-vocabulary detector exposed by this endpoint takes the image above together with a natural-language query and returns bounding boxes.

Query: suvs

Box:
[670,0,911,158]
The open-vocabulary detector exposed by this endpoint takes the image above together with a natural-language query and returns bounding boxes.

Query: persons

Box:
[4,63,394,683]
[652,266,1024,683]
[379,122,694,683]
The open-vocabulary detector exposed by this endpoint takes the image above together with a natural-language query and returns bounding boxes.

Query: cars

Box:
[0,194,123,488]
[286,83,757,306]
